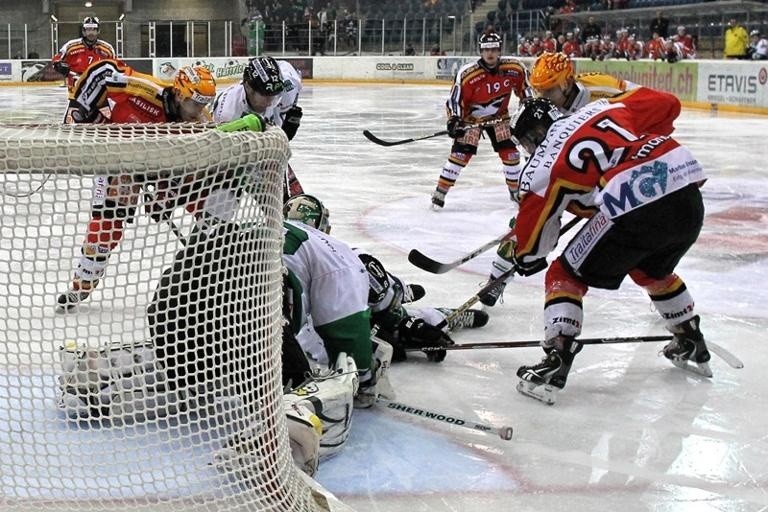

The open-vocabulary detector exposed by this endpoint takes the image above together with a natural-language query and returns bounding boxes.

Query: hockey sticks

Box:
[409,233,513,273]
[408,336,744,370]
[363,113,517,146]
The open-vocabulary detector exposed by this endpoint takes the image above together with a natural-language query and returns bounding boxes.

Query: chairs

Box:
[250,3,484,56]
[463,1,764,57]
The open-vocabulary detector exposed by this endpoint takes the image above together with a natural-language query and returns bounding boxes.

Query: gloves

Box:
[446,116,466,139]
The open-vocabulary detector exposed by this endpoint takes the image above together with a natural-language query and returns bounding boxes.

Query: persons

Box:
[517,0,768,64]
[54,16,305,313]
[431,32,536,207]
[510,87,711,389]
[240,1,487,56]
[56,194,488,512]
[529,52,640,114]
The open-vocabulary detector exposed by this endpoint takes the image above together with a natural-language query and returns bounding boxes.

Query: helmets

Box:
[509,96,562,144]
[477,32,504,52]
[172,65,218,106]
[80,16,100,36]
[243,56,285,96]
[284,195,329,233]
[530,51,575,93]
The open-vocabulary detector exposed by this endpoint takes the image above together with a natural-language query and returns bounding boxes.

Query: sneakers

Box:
[662,327,712,364]
[402,283,426,303]
[477,271,506,307]
[435,306,491,332]
[57,282,98,306]
[517,350,575,390]
[431,185,449,206]
